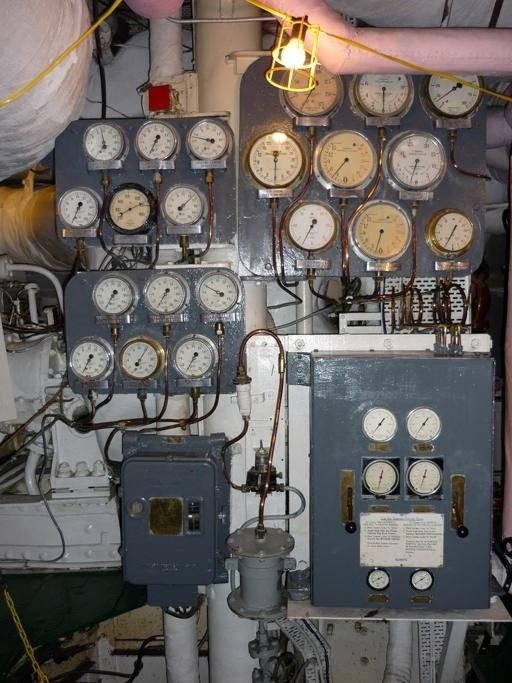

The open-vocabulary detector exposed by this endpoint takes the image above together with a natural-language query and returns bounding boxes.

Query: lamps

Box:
[265,12,319,90]
